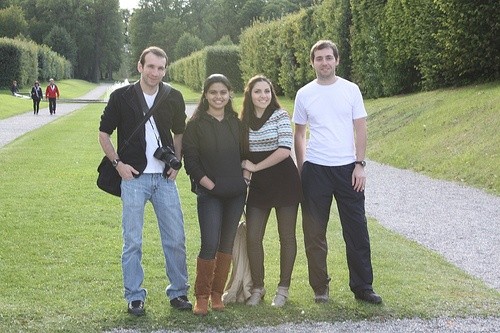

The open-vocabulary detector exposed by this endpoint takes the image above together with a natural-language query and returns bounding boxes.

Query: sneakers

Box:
[129,299,146,317]
[169,294,193,312]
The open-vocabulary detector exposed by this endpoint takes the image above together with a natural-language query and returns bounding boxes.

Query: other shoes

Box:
[37,112,38,113]
[34,112,35,114]
[271,283,291,308]
[353,288,383,305]
[50,112,52,115]
[313,280,331,302]
[246,287,266,306]
[54,112,56,114]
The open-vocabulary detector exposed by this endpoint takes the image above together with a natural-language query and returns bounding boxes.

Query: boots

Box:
[194,252,231,314]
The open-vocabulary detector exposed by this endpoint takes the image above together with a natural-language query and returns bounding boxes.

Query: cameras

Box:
[153,146,182,170]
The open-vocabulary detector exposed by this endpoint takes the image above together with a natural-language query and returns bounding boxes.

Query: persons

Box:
[240,74,305,308]
[96,46,192,315]
[292,40,382,303]
[46,78,59,115]
[31,80,43,114]
[11,81,19,95]
[182,73,252,315]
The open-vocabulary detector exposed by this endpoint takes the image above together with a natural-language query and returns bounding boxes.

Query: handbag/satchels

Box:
[95,153,124,197]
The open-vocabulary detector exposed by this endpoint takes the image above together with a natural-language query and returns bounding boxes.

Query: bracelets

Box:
[243,177,250,185]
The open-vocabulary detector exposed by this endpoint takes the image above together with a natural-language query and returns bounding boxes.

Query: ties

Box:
[52,84,53,88]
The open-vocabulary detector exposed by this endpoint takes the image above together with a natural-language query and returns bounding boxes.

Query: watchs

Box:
[112,159,121,168]
[355,160,366,167]
